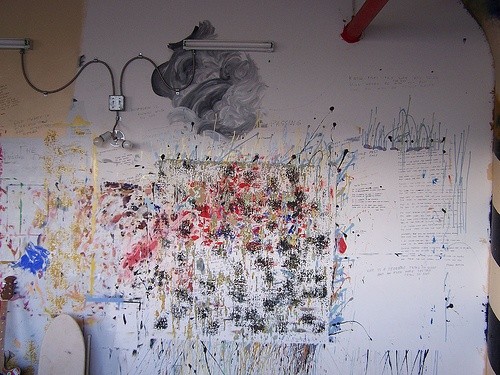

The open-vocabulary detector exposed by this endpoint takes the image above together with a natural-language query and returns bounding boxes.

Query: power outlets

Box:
[109,96,125,111]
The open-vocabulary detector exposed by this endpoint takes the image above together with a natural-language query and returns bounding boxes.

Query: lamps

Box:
[0,38,32,50]
[94,131,112,145]
[183,40,274,52]
[118,139,133,148]
